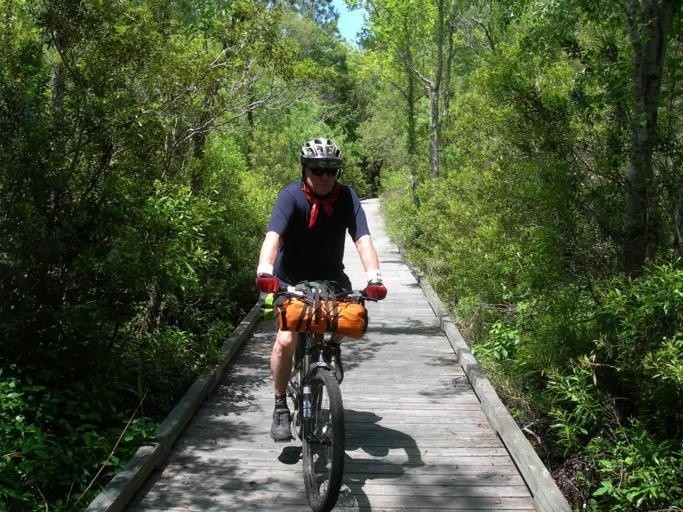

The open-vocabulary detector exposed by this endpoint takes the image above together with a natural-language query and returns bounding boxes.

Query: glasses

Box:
[304,167,339,178]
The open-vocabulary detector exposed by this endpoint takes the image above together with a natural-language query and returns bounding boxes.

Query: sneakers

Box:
[319,335,343,384]
[269,405,292,441]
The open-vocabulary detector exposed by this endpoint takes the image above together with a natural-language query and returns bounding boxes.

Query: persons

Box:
[255,139,387,440]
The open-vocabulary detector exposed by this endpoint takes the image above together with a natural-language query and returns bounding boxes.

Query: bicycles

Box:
[273,280,378,511]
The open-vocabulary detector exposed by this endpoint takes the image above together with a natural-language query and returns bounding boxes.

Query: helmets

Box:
[300,138,343,166]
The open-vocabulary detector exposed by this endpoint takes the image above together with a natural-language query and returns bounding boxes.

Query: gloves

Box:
[255,272,280,293]
[364,278,387,300]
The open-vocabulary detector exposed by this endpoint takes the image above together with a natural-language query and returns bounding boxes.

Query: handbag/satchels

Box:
[272,292,368,338]
